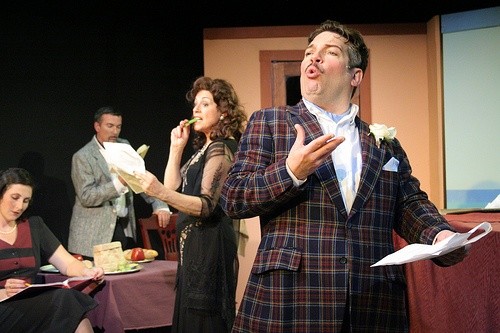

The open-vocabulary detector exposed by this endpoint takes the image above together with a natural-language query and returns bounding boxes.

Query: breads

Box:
[123,249,158,259]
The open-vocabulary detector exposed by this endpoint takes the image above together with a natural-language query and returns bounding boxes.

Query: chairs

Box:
[138,214,180,262]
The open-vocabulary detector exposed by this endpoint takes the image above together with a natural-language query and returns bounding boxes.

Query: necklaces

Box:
[0,224,17,234]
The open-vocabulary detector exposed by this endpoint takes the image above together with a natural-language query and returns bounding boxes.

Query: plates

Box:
[40,264,60,272]
[127,258,155,263]
[104,264,144,274]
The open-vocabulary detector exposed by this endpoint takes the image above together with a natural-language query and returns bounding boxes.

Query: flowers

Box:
[369,123,397,149]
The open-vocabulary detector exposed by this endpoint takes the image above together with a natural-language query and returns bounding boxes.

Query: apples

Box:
[131,248,145,261]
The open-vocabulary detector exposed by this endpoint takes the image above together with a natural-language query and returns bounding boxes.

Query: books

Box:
[110,144,150,175]
[1,275,95,304]
[99,143,146,194]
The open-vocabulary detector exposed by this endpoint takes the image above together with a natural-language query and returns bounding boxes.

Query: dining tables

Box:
[45,260,179,333]
[392,212,500,333]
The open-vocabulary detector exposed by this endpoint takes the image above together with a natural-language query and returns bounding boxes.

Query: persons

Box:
[220,23,472,333]
[66,106,171,262]
[133,77,250,333]
[0,168,105,333]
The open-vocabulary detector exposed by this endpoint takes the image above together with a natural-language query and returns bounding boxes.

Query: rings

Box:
[140,179,145,185]
